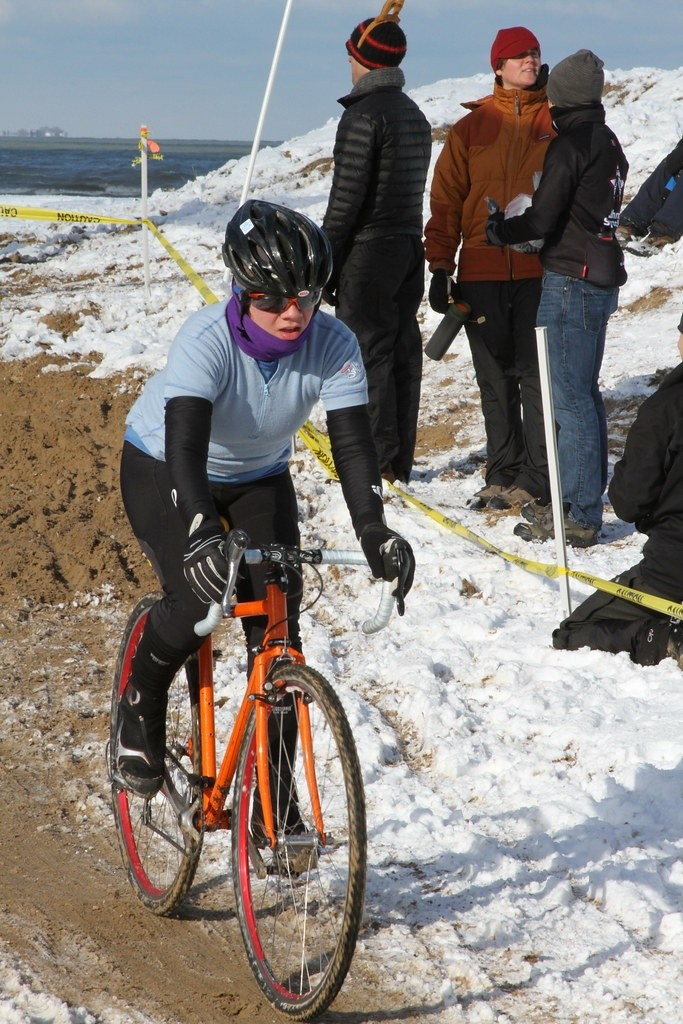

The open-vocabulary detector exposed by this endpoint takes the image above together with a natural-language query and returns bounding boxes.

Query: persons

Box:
[321,15,431,485]
[552,313,683,668]
[115,198,416,880]
[483,48,628,547]
[613,136,683,257]
[423,27,559,512]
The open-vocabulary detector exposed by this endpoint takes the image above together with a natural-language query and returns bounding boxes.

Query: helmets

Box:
[221,200,333,298]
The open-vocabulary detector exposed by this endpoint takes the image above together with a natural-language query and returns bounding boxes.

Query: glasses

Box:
[241,286,325,313]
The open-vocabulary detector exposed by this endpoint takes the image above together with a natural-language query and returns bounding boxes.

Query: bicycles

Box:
[105,526,407,1022]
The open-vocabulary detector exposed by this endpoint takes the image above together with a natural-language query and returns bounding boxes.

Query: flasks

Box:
[424,300,472,361]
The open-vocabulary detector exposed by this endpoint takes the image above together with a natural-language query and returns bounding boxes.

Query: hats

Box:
[345,18,407,69]
[491,27,541,72]
[546,49,604,109]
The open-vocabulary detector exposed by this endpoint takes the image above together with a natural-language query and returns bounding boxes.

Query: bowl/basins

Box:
[503,194,544,247]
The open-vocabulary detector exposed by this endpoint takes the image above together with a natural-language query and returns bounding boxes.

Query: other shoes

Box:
[662,601,683,672]
[381,470,398,503]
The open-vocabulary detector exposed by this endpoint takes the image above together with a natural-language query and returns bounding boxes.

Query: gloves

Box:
[359,522,416,600]
[320,278,338,306]
[485,211,505,245]
[428,272,459,313]
[181,525,247,605]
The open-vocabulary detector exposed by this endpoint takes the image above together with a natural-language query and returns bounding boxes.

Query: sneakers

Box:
[514,515,600,548]
[626,232,675,257]
[113,676,169,796]
[490,482,534,511]
[522,498,570,523]
[465,482,510,510]
[250,796,321,867]
[615,224,641,248]
